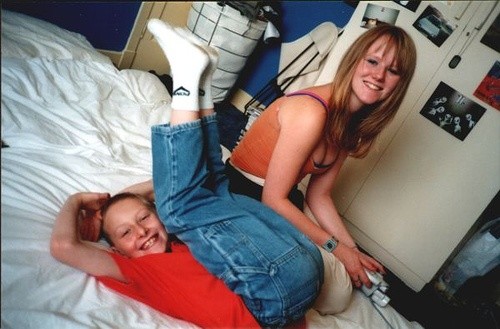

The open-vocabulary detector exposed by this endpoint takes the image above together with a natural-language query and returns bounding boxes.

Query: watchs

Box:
[321,236,339,253]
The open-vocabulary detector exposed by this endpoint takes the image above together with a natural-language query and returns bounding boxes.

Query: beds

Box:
[0,11,423,329]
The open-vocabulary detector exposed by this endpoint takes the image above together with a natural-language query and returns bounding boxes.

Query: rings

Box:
[353,278,360,282]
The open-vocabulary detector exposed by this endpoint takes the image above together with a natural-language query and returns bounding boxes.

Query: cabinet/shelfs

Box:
[296,0,500,293]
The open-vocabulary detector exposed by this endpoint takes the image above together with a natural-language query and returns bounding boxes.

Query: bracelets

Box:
[352,245,358,250]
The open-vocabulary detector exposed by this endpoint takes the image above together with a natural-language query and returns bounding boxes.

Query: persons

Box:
[224,25,418,319]
[49,18,326,329]
